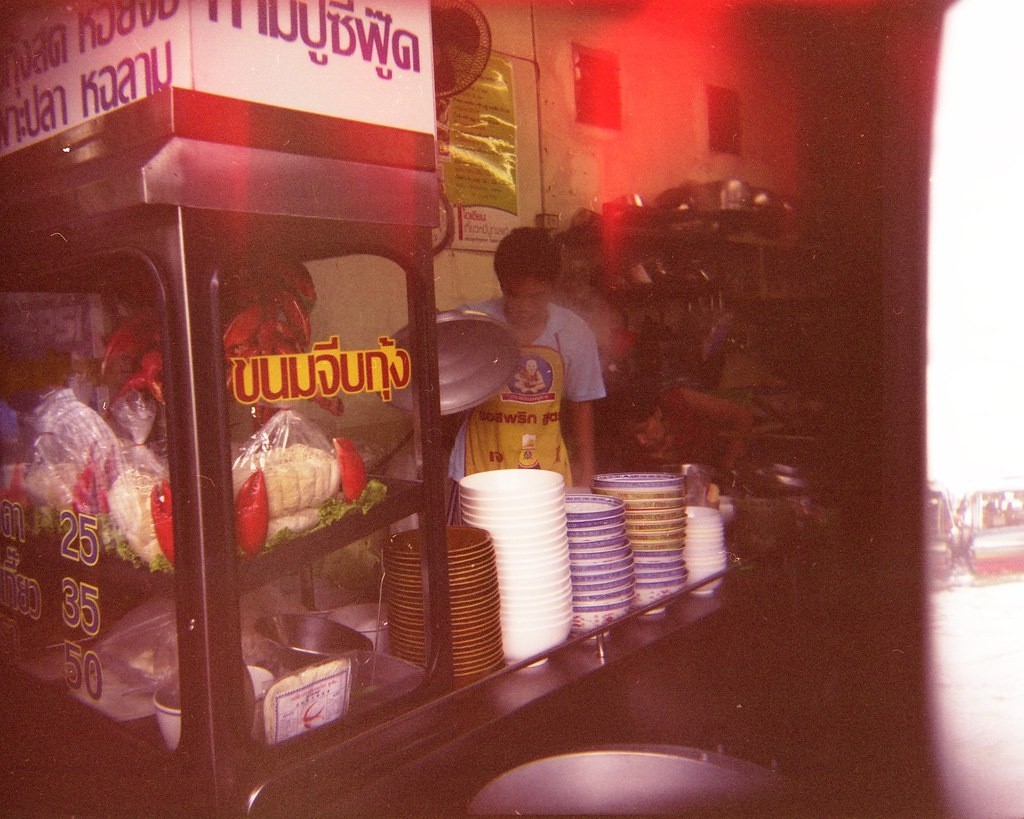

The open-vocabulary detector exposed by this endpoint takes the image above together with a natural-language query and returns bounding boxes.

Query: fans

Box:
[430,0,492,116]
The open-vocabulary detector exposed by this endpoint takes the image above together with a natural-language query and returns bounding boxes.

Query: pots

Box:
[615,178,795,215]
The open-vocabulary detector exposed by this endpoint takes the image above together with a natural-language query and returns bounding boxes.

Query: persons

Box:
[445,224,606,490]
[601,367,754,478]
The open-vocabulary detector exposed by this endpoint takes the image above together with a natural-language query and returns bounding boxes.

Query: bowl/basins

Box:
[152,468,728,749]
